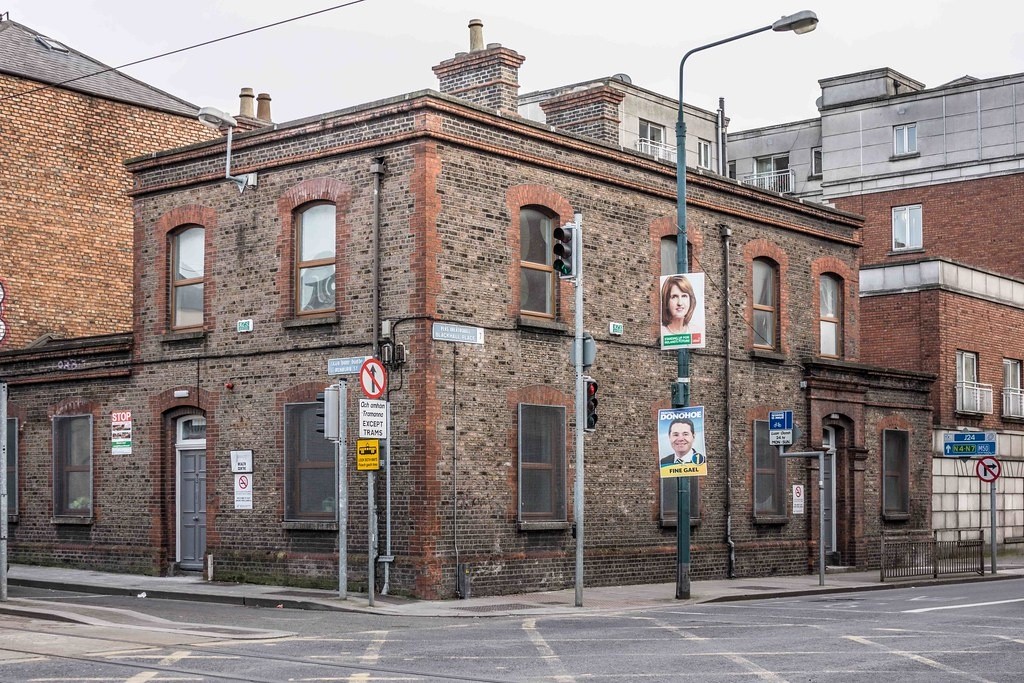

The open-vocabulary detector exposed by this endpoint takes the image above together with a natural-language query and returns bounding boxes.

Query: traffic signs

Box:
[942,431,997,443]
[943,442,996,457]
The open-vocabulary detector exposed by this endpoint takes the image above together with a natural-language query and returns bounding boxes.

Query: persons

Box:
[661,417,706,464]
[661,275,696,334]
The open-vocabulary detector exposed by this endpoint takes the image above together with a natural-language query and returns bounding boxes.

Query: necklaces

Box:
[666,324,688,333]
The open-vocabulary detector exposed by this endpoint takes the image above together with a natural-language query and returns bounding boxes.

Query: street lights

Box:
[675,10,819,599]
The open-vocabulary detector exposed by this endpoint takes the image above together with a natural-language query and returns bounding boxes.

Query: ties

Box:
[676,459,682,463]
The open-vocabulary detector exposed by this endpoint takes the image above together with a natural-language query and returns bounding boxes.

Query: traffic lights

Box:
[550,224,576,282]
[581,376,598,431]
